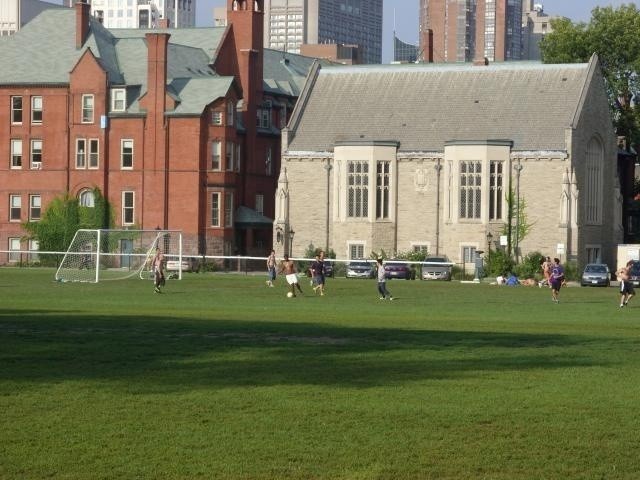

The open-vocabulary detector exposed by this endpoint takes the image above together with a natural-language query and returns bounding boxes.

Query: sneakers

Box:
[266,281,274,287]
[313,286,325,296]
[390,297,392,300]
[552,296,559,301]
[380,297,386,299]
[154,288,160,293]
[620,302,627,309]
[538,281,542,288]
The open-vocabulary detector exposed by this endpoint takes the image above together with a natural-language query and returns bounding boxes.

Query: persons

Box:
[79,246,86,271]
[497,256,566,288]
[615,260,636,307]
[154,254,165,293]
[151,248,161,288]
[266,250,277,287]
[310,251,325,291]
[310,255,325,296]
[550,258,564,303]
[376,260,394,301]
[277,255,303,297]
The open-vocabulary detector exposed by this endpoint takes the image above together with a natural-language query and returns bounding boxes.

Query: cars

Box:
[628,260,640,288]
[422,255,453,281]
[383,262,416,280]
[167,257,200,273]
[308,262,332,277]
[581,264,611,286]
[345,262,375,279]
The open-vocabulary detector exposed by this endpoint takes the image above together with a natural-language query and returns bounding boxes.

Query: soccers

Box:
[287,292,293,297]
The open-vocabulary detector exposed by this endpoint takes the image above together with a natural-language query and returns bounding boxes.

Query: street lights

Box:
[486,232,493,276]
[153,226,161,249]
[288,227,295,257]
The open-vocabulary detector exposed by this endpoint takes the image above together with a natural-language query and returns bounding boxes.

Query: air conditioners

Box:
[32,163,39,169]
